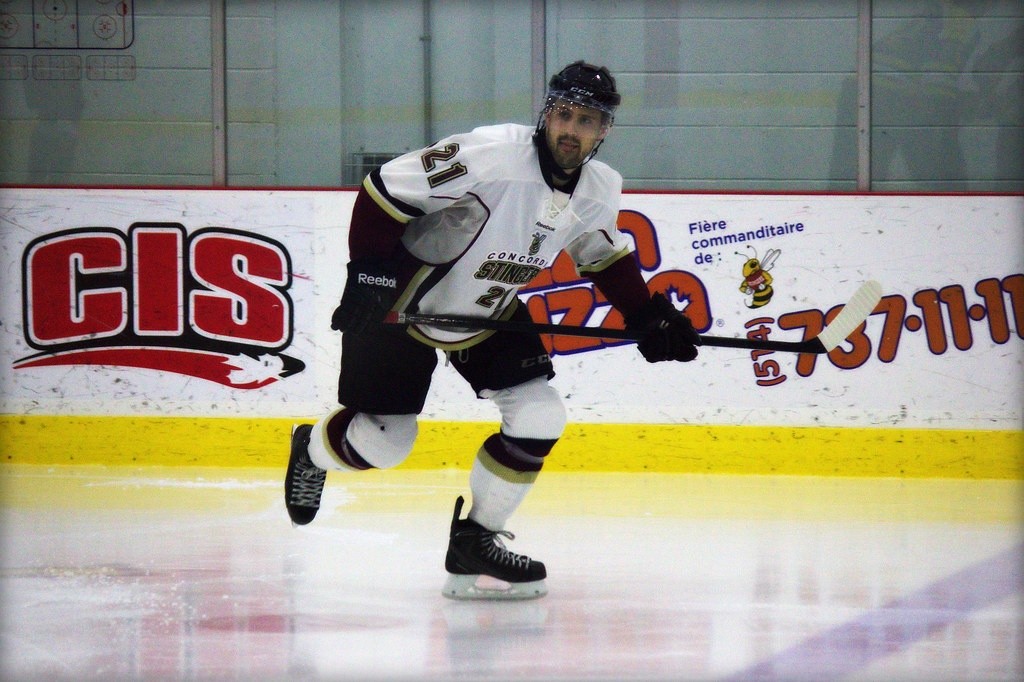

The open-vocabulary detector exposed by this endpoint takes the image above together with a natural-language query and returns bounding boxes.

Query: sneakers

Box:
[284,424,327,528]
[440,495,549,602]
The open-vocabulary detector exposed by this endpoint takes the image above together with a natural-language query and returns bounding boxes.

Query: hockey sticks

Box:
[382,280,884,354]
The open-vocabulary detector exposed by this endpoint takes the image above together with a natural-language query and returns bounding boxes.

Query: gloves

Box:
[330,256,403,336]
[624,291,701,363]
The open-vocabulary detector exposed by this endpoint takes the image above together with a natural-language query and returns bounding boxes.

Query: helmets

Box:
[544,59,621,117]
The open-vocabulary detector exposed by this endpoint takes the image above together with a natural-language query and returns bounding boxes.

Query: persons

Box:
[285,61,698,600]
[826,1,1024,192]
